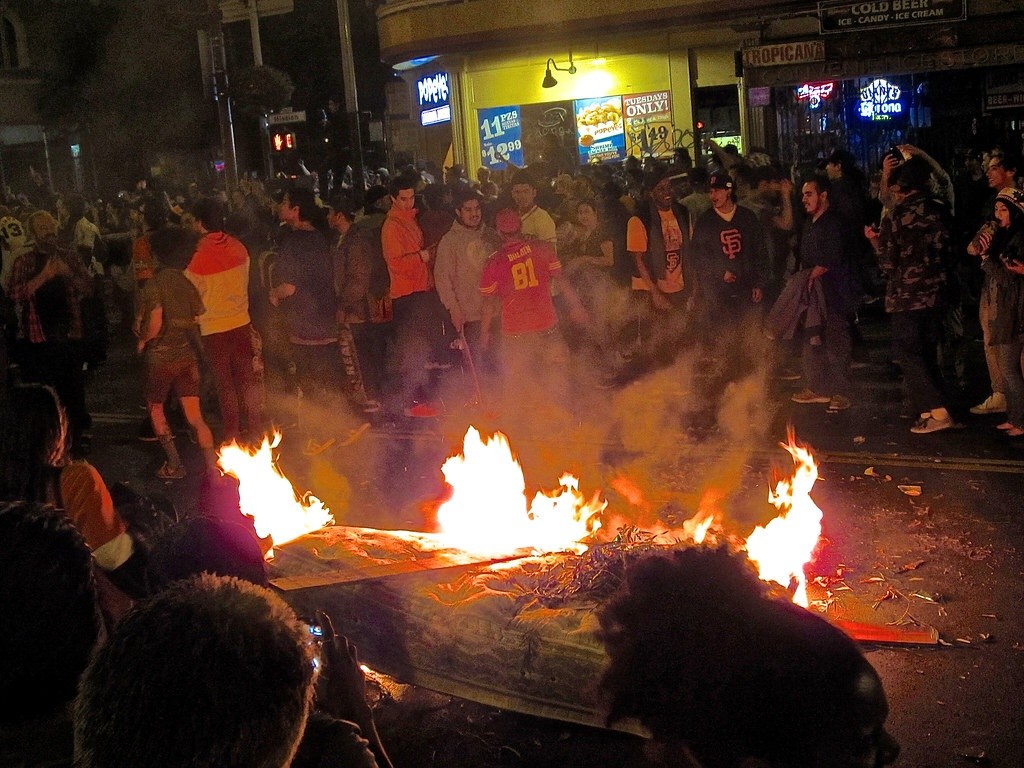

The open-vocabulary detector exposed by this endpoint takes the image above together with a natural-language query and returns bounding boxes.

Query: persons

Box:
[790,180,854,413]
[0,499,103,768]
[183,198,265,456]
[142,512,393,768]
[594,543,900,768]
[0,135,1024,484]
[0,384,152,630]
[74,576,321,768]
[478,208,588,426]
[268,187,371,456]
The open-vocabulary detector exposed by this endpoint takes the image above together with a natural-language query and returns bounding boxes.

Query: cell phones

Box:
[889,147,900,165]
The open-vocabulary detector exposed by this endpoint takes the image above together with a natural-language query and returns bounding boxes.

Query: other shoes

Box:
[338,423,371,447]
[137,426,176,441]
[900,409,917,419]
[302,436,336,456]
[404,403,442,418]
[154,462,185,479]
[989,420,1024,441]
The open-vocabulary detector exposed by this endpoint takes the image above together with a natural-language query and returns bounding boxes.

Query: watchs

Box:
[334,706,374,722]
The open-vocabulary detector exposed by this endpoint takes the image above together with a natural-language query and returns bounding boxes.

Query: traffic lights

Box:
[271,132,284,151]
[285,132,296,150]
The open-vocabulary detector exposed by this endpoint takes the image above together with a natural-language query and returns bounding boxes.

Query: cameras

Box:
[294,617,324,673]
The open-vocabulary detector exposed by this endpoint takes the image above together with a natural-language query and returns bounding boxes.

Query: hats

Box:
[496,209,521,233]
[644,169,668,190]
[707,174,732,186]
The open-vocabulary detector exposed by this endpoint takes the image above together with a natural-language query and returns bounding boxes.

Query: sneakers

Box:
[792,389,831,403]
[826,395,853,414]
[910,414,953,433]
[970,392,1008,414]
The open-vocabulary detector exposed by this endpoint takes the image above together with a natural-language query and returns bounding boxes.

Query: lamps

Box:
[542,57,577,88]
